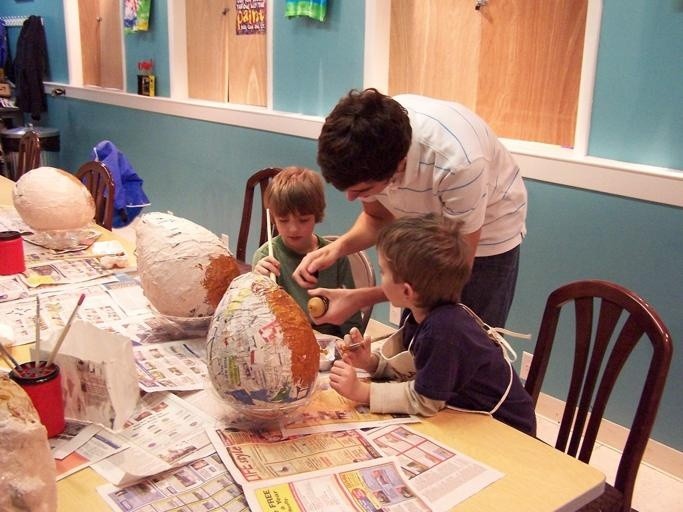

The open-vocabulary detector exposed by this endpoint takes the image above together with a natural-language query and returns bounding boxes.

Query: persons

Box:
[293,88,528,346]
[329,212,536,441]
[252,166,364,338]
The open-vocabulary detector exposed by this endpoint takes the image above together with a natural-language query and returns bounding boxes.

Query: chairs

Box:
[12,130,40,182]
[71,163,117,231]
[317,233,377,339]
[512,276,674,512]
[231,165,282,275]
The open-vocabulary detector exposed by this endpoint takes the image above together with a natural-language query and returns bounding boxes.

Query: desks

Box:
[1,173,606,512]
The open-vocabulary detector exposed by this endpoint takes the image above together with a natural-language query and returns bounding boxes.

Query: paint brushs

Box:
[35,296,40,369]
[46,293,86,368]
[266,208,276,284]
[346,332,392,352]
[0,344,24,378]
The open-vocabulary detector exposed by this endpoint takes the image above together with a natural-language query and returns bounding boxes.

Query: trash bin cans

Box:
[3,122,60,182]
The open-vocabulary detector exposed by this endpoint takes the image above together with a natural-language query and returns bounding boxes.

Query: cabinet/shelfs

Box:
[386,0,587,150]
[184,0,268,108]
[76,0,123,91]
